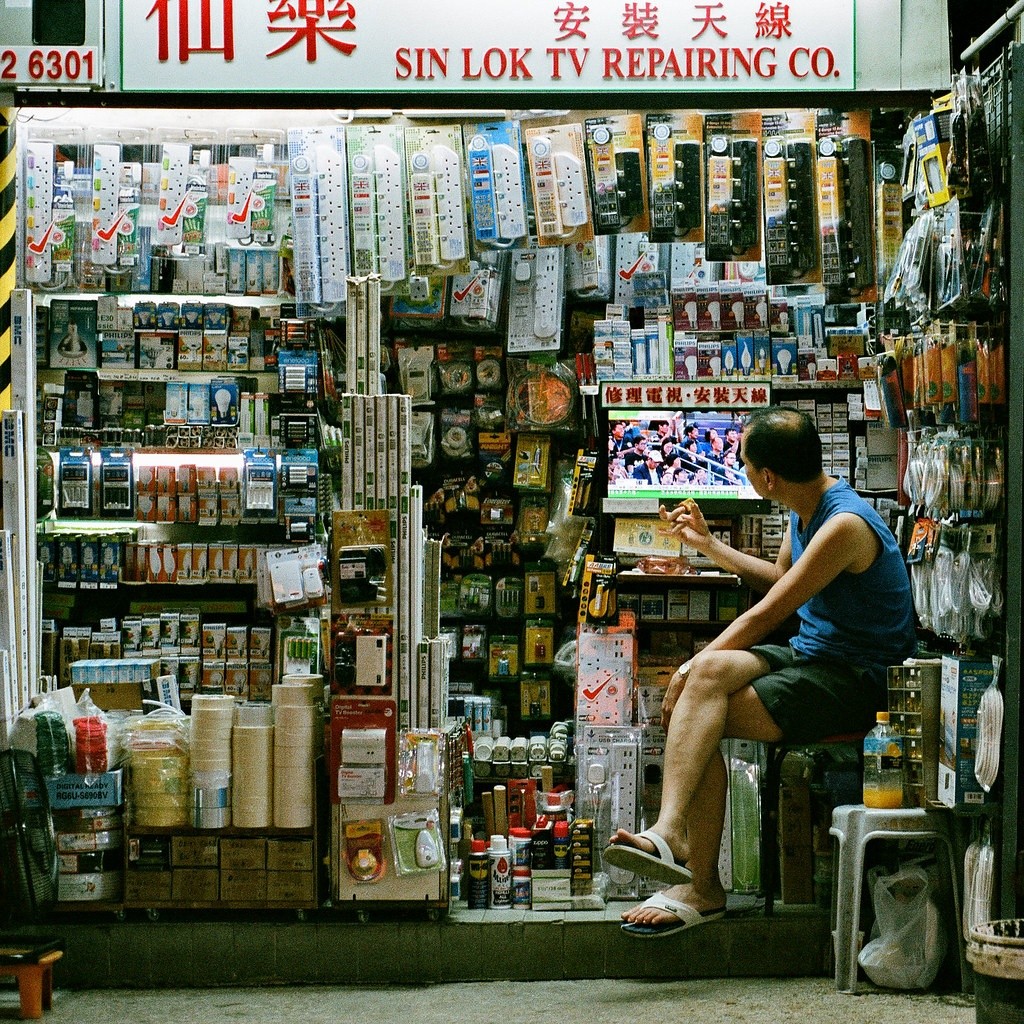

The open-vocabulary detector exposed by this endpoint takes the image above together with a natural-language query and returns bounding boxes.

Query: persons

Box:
[603,405,920,942]
[607,416,753,488]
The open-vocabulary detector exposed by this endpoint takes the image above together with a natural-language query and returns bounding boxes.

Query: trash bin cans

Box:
[965,919,1024,1024]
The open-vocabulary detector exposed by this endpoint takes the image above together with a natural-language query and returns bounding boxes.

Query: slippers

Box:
[603,831,693,884]
[619,891,727,940]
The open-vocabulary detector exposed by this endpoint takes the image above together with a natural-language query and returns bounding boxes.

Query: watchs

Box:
[676,662,692,682]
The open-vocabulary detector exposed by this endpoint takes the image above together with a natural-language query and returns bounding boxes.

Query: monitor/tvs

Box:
[602,406,772,517]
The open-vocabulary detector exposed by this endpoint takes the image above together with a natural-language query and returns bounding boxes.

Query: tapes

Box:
[232,703,274,828]
[127,718,188,827]
[188,693,234,828]
[272,674,323,829]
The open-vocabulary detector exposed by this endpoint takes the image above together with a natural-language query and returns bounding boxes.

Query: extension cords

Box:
[574,634,636,886]
[717,758,733,894]
[32,115,875,338]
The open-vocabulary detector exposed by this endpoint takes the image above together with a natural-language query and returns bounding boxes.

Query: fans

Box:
[0,748,63,966]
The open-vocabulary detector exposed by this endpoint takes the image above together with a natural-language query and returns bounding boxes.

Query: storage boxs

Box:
[531,819,595,911]
[220,837,266,870]
[138,466,240,523]
[172,869,219,902]
[50,295,281,371]
[938,656,994,809]
[165,378,238,425]
[172,836,218,866]
[19,768,125,809]
[268,838,313,870]
[220,869,266,901]
[173,242,279,294]
[57,807,121,901]
[616,393,899,620]
[40,593,271,699]
[595,280,876,379]
[36,526,308,585]
[267,871,314,903]
[126,871,171,902]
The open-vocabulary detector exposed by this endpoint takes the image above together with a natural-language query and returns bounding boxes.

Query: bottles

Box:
[460,824,472,901]
[508,827,526,862]
[467,840,490,909]
[513,866,531,909]
[514,831,533,866]
[863,712,903,808]
[554,820,568,870]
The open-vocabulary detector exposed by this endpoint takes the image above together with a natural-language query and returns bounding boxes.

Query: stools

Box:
[757,727,870,916]
[0,948,62,1019]
[829,803,973,997]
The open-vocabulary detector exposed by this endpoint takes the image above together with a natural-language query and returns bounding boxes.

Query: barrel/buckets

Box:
[966,918,1024,1024]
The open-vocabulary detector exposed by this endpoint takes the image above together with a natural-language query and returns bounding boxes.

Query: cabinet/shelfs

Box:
[33,291,355,701]
[886,664,941,807]
[602,380,863,633]
